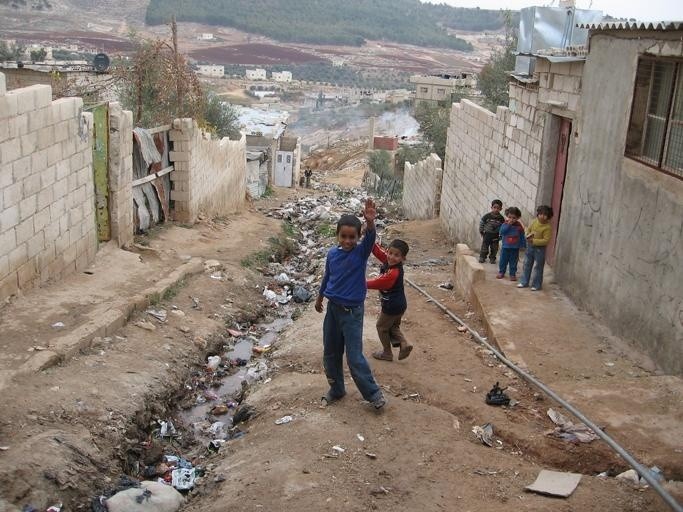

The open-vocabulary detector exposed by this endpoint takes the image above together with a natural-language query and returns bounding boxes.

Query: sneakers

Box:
[496,272,504,278]
[517,283,526,287]
[510,275,516,281]
[531,286,542,290]
[398,338,413,360]
[373,351,393,361]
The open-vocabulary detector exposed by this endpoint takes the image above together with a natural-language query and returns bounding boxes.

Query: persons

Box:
[496,207,526,281]
[517,205,553,291]
[314,198,385,409]
[304,166,313,184]
[361,224,412,361]
[479,199,505,264]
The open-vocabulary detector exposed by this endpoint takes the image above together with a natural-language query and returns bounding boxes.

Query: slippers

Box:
[374,398,386,410]
[321,392,337,406]
